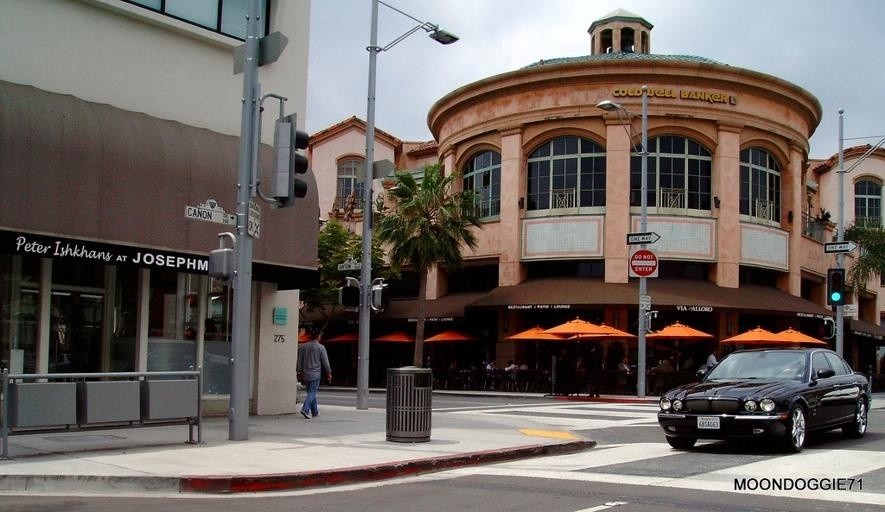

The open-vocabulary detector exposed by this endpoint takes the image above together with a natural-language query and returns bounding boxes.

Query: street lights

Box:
[824,241,858,254]
[597,85,648,398]
[357,0,460,410]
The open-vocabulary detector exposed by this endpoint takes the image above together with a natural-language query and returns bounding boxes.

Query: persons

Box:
[487,358,528,389]
[292,328,332,419]
[705,348,718,373]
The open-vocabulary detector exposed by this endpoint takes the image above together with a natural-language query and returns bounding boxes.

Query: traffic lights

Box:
[275,114,309,208]
[827,269,846,306]
[373,283,390,308]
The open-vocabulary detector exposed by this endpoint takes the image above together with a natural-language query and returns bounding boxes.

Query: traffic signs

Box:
[625,233,661,245]
[187,200,237,226]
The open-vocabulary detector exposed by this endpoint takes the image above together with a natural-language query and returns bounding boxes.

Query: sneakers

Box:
[301,411,320,418]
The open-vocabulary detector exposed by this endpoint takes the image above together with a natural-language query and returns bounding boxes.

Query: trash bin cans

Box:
[386,366,432,443]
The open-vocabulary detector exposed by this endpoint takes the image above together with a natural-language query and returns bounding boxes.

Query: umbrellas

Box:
[719,324,792,349]
[504,316,636,397]
[775,326,828,346]
[645,321,716,371]
[326,332,358,345]
[373,330,414,345]
[424,330,474,345]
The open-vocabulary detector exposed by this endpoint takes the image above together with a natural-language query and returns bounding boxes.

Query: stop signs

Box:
[629,249,658,277]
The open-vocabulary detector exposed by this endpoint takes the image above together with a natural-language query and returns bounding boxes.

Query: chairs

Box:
[442,369,678,396]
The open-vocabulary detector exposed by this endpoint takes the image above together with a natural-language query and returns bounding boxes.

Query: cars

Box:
[658,347,869,452]
